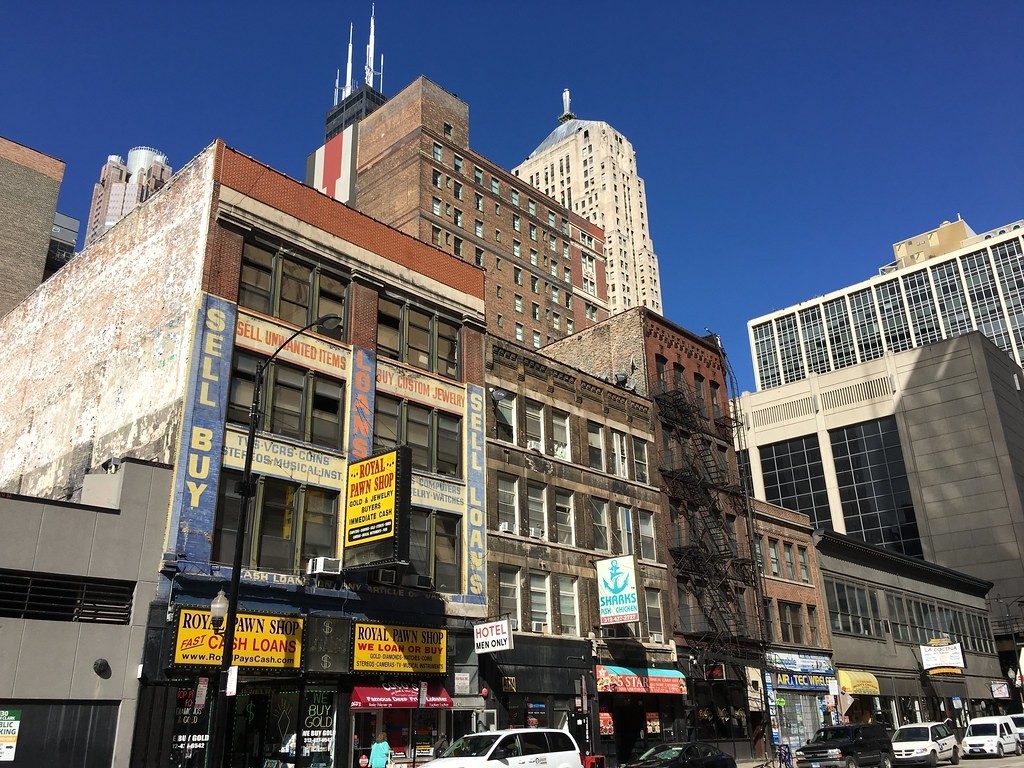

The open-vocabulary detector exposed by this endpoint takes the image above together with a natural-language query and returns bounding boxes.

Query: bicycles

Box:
[772,744,793,768]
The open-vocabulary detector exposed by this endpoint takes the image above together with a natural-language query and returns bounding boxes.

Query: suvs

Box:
[795,722,896,768]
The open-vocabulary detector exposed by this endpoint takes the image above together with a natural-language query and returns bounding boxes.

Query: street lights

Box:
[202,311,342,768]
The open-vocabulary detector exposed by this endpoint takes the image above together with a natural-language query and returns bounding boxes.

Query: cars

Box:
[625,741,737,768]
[415,727,588,768]
[891,717,960,767]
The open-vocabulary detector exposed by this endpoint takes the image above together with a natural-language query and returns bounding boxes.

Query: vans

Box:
[1006,713,1024,755]
[962,715,1023,759]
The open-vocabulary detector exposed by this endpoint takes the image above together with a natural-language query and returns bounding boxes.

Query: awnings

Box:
[596,664,688,694]
[349,681,454,711]
[837,670,880,695]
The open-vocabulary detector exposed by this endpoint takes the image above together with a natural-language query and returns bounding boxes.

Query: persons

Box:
[432,733,451,761]
[367,731,393,768]
